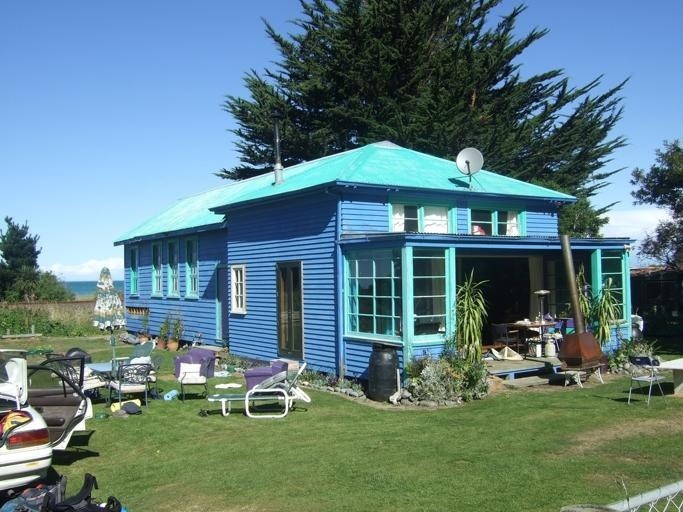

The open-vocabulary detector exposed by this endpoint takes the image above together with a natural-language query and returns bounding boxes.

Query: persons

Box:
[472,225,486,235]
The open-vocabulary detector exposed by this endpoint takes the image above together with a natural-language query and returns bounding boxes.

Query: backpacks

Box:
[122,402,143,416]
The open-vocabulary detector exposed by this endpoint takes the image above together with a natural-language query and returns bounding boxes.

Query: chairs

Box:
[485,323,539,360]
[174,348,215,403]
[625,355,667,406]
[205,359,307,419]
[45,340,156,412]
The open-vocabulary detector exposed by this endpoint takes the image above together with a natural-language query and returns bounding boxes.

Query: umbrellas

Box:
[92,266,128,358]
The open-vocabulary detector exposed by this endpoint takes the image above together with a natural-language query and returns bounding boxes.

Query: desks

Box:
[515,320,558,356]
[643,358,683,398]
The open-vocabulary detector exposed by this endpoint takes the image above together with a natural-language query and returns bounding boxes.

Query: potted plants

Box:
[137,308,184,351]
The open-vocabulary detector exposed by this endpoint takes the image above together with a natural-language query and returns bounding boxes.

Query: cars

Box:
[1,348,88,496]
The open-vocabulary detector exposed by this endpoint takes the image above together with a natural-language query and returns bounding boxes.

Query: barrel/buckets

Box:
[368,343,399,402]
[544,343,556,357]
